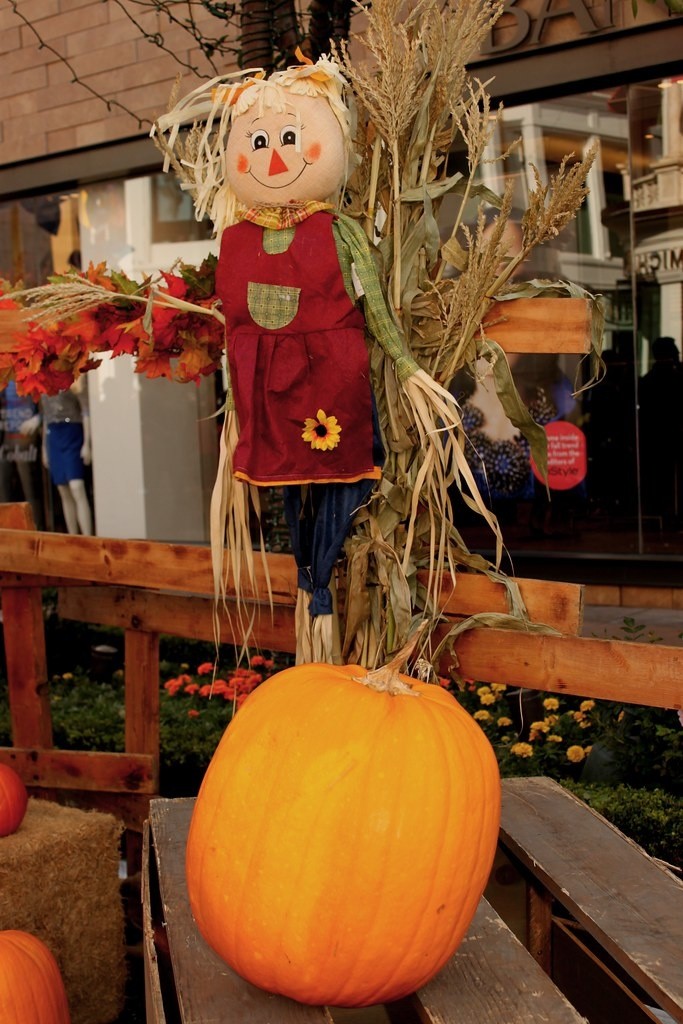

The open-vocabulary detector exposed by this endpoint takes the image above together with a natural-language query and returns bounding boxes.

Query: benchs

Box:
[131,768,682,1020]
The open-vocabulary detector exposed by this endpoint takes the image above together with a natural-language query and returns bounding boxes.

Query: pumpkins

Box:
[0,763,28,836]
[0,932,71,1024]
[184,619,502,1009]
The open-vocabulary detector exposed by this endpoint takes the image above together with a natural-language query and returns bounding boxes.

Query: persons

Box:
[638,335,683,535]
[32,371,99,536]
[11,38,482,664]
[458,350,565,527]
[517,484,576,539]
[603,338,651,526]
